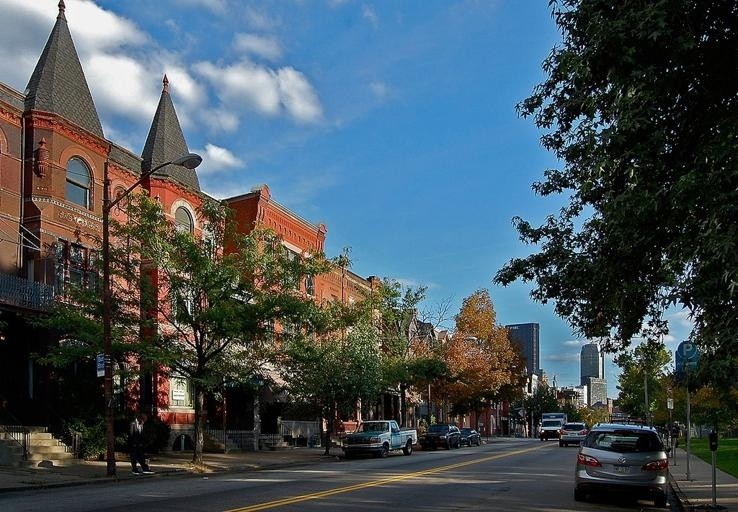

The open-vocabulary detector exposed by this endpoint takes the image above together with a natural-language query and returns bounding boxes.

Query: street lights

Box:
[428,336,476,426]
[100,152,202,474]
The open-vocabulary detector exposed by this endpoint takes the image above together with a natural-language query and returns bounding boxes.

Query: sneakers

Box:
[143,470,155,475]
[132,470,140,475]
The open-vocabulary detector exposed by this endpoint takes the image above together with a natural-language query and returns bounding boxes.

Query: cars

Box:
[558,422,590,448]
[573,422,670,508]
[459,428,480,447]
[418,423,461,451]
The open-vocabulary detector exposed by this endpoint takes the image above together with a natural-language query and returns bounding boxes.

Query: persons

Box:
[126,413,156,475]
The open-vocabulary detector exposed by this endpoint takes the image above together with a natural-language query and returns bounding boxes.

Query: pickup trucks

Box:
[342,420,418,460]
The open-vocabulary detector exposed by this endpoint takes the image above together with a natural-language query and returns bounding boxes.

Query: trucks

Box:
[540,413,566,441]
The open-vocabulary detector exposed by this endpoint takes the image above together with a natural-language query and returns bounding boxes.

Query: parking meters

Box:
[657,427,677,467]
[707,430,717,504]
